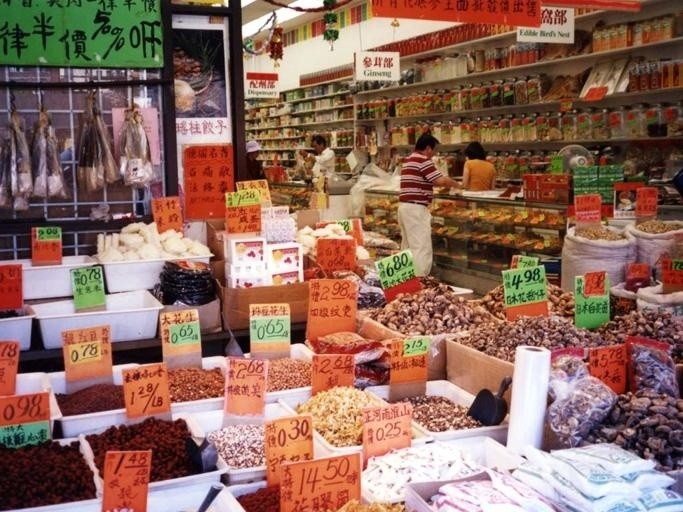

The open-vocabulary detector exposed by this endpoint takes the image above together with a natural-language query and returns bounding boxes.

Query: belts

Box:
[405,200,429,207]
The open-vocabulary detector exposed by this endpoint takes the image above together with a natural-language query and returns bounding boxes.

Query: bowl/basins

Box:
[92,252,213,293]
[31,290,163,349]
[79,413,228,495]
[15,372,61,438]
[194,403,328,484]
[171,356,226,412]
[48,364,141,436]
[365,380,508,444]
[243,343,318,401]
[0,255,95,299]
[0,482,246,512]
[0,305,37,351]
[228,480,266,496]
[277,391,432,453]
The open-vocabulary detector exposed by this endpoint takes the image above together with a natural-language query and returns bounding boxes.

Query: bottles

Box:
[450,74,547,110]
[390,112,562,146]
[356,89,449,119]
[486,149,557,178]
[561,100,682,139]
[432,151,464,177]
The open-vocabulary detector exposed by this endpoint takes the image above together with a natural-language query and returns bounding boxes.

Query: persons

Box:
[299,135,335,181]
[242,139,266,182]
[462,141,496,190]
[397,133,462,278]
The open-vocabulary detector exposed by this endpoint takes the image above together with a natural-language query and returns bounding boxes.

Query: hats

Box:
[246,140,263,153]
